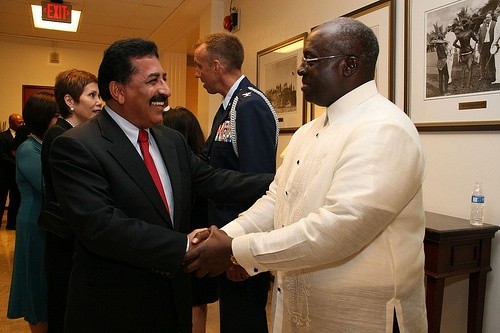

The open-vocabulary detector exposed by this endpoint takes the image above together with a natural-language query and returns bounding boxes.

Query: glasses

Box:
[296,54,358,72]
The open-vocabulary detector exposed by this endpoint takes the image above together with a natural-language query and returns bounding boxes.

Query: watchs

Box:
[229,251,239,267]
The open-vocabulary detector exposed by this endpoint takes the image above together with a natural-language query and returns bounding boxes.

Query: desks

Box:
[423,211,500,333]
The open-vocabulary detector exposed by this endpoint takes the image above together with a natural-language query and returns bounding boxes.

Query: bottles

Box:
[470,182,486,227]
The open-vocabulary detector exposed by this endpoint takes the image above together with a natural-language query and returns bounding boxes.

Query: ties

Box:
[138,130,171,216]
[211,103,224,132]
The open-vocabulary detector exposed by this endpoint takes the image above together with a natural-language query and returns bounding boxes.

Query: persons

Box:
[182,16,428,333]
[429,3,500,96]
[0,32,279,333]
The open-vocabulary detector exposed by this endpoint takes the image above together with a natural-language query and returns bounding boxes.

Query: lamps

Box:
[41,1,72,23]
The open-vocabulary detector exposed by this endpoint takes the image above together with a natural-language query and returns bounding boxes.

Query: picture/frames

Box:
[256,31,308,135]
[309,0,397,121]
[403,0,500,134]
